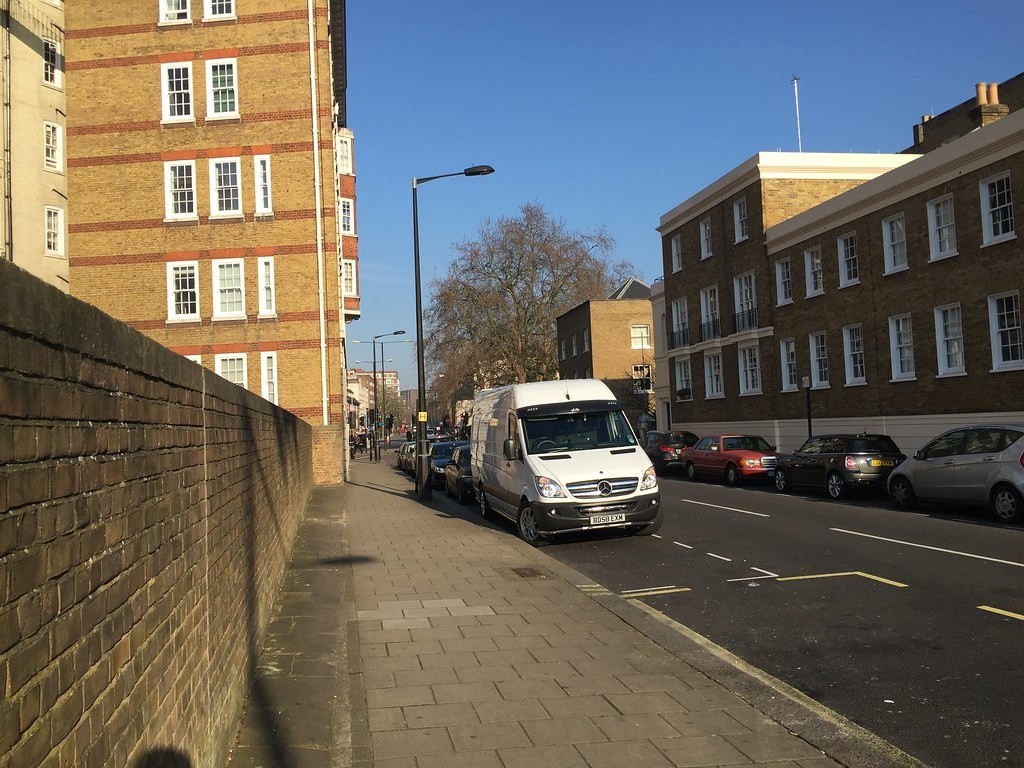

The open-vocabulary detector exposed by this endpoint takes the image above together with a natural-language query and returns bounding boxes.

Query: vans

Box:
[470,377,663,550]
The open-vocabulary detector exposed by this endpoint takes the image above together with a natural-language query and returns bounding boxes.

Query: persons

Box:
[353,434,363,455]
[406,430,412,441]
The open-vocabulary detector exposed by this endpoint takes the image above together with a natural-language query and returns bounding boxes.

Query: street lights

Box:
[373,330,406,462]
[351,340,413,461]
[354,359,395,456]
[411,165,496,503]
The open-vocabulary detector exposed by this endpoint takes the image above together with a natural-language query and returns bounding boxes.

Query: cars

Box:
[393,428,455,479]
[771,433,909,499]
[428,441,470,491]
[680,433,796,488]
[444,444,473,504]
[887,424,1024,524]
[634,430,700,476]
